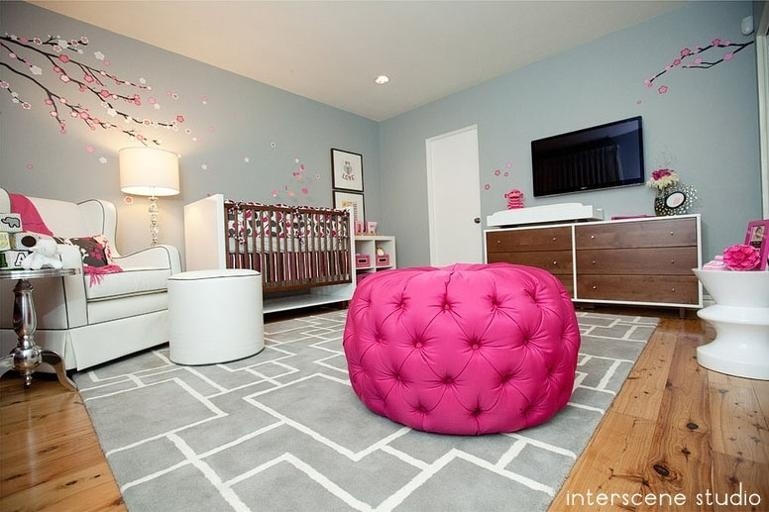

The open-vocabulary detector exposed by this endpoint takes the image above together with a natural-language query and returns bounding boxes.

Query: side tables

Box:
[0,266,83,391]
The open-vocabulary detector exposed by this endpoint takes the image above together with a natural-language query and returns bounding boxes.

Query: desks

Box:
[482,215,704,310]
[689,267,769,384]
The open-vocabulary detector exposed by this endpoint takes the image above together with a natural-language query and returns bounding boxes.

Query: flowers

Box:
[647,169,681,189]
[722,244,759,273]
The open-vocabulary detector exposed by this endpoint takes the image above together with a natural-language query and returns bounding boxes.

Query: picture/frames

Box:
[330,149,366,190]
[333,191,367,232]
[742,218,769,270]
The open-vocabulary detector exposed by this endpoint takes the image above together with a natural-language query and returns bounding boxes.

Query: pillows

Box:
[52,233,123,287]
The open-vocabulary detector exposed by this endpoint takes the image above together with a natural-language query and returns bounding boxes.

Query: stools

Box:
[167,266,266,366]
[343,263,579,435]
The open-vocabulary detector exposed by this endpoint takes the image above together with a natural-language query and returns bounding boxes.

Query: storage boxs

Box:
[356,255,371,267]
[356,273,369,286]
[376,255,389,266]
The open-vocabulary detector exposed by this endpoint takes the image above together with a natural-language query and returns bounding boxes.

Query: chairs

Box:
[0,188,181,371]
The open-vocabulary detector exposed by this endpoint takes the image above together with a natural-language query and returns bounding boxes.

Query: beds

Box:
[224,201,352,295]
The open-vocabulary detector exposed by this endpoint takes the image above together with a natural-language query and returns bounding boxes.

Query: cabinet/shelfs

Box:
[355,236,398,270]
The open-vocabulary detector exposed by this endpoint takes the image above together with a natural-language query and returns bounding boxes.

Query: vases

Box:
[653,191,668,214]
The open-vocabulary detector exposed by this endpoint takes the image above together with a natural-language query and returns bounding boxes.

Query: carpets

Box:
[74,309,658,512]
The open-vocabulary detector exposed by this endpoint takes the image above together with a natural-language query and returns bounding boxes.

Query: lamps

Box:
[117,145,181,246]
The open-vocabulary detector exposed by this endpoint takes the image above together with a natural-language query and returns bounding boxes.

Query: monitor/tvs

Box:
[531,115,644,199]
